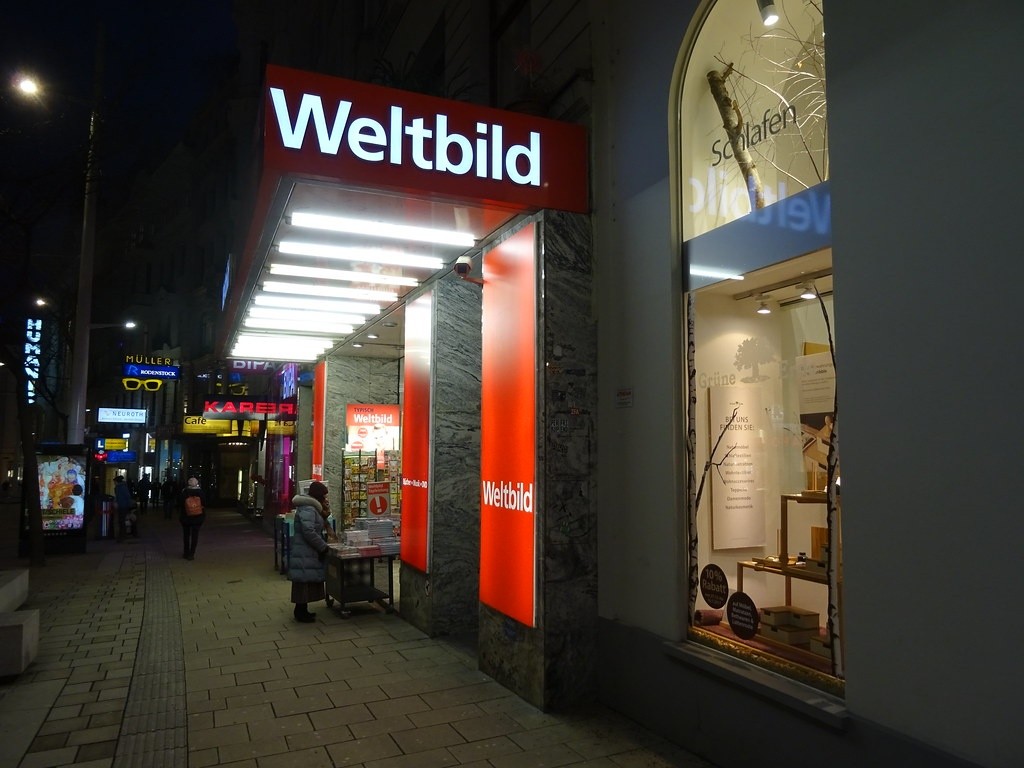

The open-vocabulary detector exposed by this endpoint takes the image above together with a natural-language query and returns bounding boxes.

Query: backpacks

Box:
[185,496,203,516]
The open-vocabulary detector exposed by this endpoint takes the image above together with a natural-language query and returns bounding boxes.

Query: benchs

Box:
[0,608,41,677]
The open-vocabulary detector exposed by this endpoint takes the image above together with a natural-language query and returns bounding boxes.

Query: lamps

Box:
[795,281,817,300]
[753,292,772,314]
[756,0,779,26]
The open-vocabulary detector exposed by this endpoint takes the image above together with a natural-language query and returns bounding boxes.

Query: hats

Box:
[113,475,124,482]
[309,481,329,500]
[188,477,199,486]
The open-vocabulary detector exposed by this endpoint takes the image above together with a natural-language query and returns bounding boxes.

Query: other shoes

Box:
[187,553,195,561]
[116,536,127,543]
[294,609,317,624]
[183,551,190,558]
[303,608,317,617]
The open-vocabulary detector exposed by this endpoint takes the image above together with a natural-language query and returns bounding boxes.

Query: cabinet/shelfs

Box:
[737,489,843,678]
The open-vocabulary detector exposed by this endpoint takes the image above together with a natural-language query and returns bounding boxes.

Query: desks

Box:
[275,514,336,576]
[325,543,400,618]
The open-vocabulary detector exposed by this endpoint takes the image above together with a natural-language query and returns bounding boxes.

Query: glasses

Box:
[122,377,163,391]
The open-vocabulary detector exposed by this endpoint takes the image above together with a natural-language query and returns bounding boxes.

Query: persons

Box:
[287,481,331,623]
[178,478,207,560]
[65,468,84,527]
[113,476,140,542]
[139,473,184,511]
